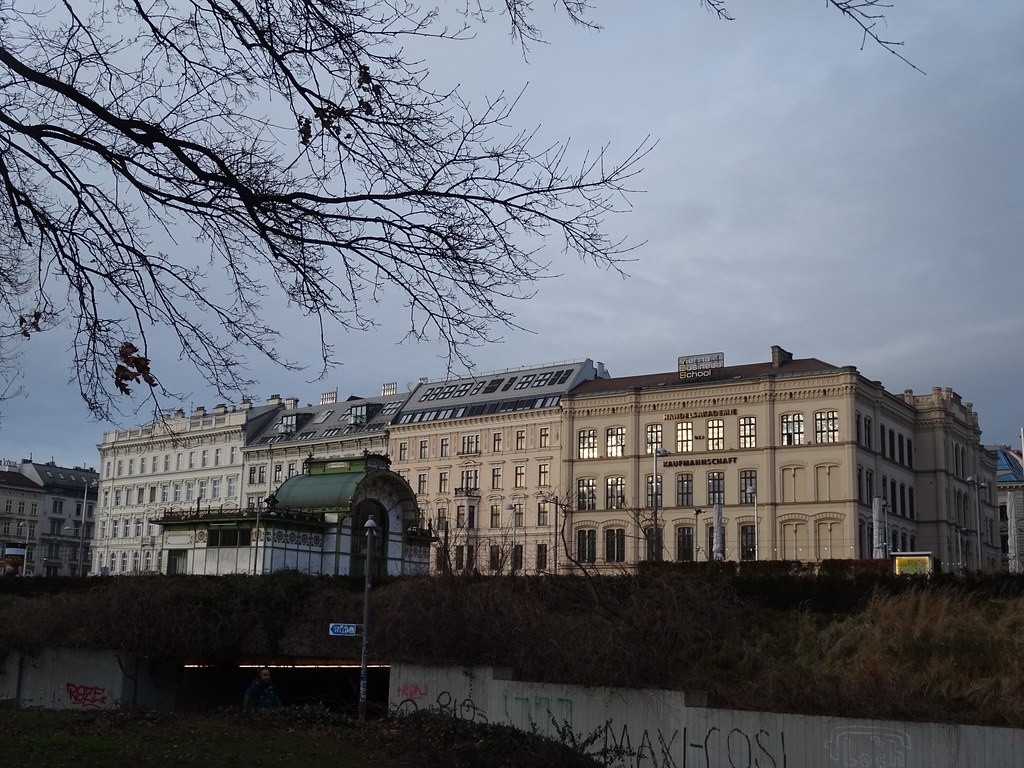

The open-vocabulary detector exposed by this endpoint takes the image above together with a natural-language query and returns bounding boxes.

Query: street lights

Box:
[357,514,378,724]
[746,488,760,560]
[538,497,558,575]
[882,502,894,559]
[240,507,260,576]
[652,446,669,562]
[134,520,145,577]
[504,503,516,576]
[967,473,988,569]
[20,521,30,578]
[64,525,85,579]
